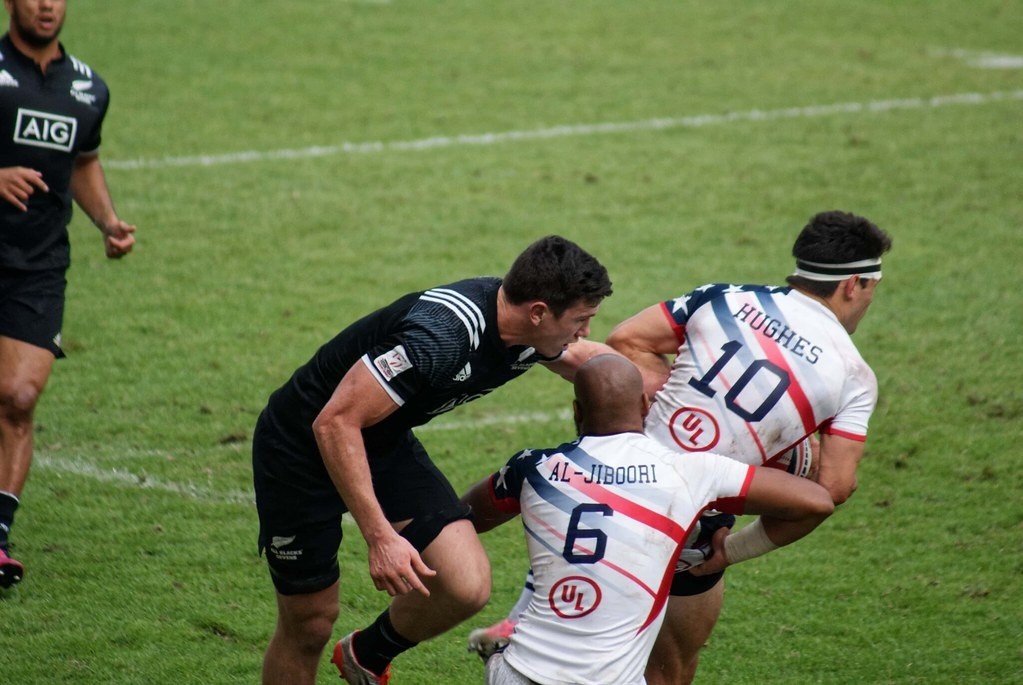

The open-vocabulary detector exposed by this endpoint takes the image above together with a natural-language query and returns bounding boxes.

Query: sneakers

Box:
[467,622,511,664]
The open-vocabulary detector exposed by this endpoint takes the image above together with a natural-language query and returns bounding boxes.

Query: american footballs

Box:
[765,432,821,482]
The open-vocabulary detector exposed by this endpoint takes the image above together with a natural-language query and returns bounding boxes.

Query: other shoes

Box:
[0,549,23,589]
[331,630,389,685]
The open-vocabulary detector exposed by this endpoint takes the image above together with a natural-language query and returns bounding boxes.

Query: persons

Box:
[0,0,136,590]
[251,236,670,685]
[468,212,893,685]
[460,353,834,685]
